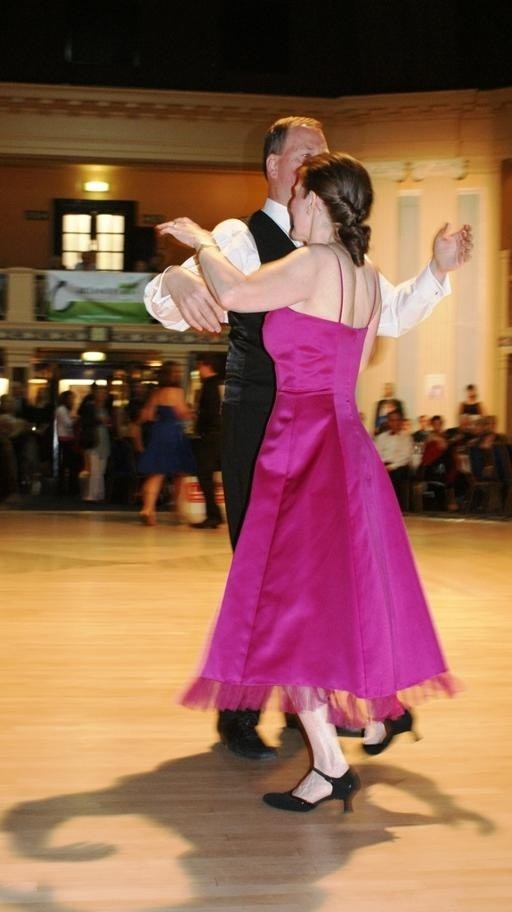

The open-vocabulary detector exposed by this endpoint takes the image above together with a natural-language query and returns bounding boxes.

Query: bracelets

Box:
[197,241,219,259]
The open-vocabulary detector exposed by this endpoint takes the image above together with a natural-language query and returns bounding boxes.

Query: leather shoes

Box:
[218,724,279,759]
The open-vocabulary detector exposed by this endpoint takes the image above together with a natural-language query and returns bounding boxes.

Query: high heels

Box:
[262,766,361,814]
[362,708,419,755]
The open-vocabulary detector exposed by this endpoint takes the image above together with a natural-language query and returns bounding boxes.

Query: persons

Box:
[4,352,226,529]
[50,281,75,313]
[156,149,460,813]
[144,116,474,759]
[372,381,511,520]
